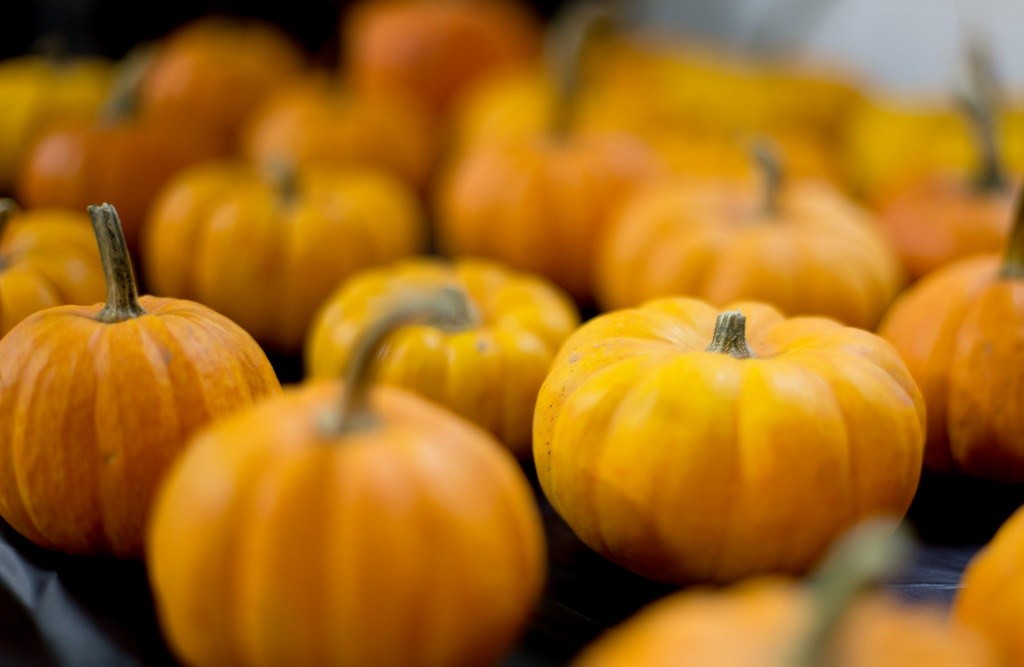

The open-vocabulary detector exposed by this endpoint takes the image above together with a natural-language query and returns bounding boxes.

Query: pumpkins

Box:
[0,0,1024,667]
